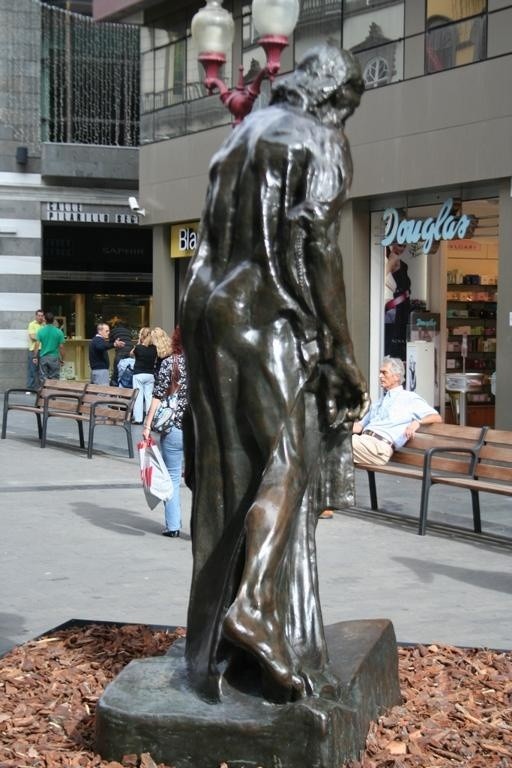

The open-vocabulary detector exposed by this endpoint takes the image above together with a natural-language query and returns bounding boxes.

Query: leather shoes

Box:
[161,528,179,537]
[319,509,333,518]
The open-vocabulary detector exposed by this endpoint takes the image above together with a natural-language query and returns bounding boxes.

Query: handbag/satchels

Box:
[138,434,174,511]
[150,378,183,437]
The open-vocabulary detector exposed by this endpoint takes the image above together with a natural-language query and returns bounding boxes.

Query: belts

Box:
[362,430,397,452]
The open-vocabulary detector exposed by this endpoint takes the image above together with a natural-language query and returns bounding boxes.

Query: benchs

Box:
[1,378,140,459]
[353,423,512,536]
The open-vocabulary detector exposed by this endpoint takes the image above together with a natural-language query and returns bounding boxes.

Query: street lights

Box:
[190,0,304,131]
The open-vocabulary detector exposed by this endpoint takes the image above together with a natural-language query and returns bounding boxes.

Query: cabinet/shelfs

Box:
[444,284,497,429]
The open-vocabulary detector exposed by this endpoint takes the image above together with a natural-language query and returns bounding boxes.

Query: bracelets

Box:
[144,425,151,430]
[416,418,422,424]
[33,356,38,359]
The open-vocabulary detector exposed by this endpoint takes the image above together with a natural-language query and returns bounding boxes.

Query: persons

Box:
[317,354,442,519]
[178,42,371,695]
[130,327,155,425]
[110,320,135,388]
[89,322,125,420]
[25,310,45,395]
[385,240,412,360]
[142,324,190,537]
[151,327,173,378]
[32,312,66,401]
